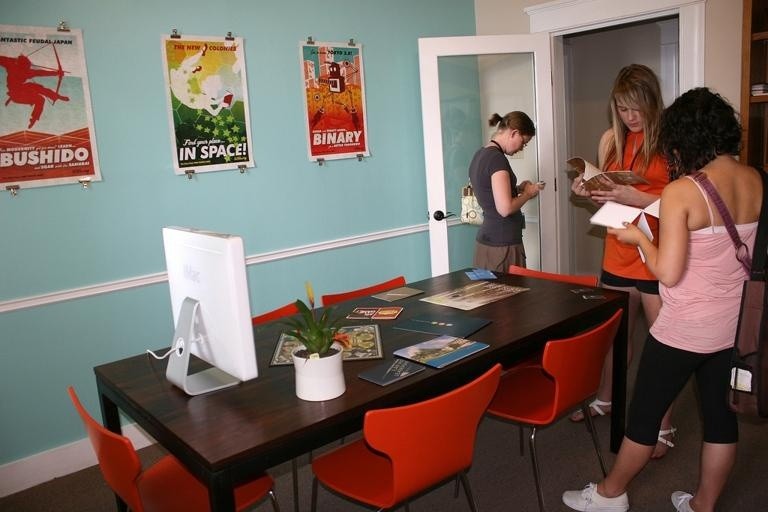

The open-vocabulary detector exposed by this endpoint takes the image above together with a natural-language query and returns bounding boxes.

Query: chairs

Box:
[322,277,406,306]
[508,265,598,456]
[454,305,625,512]
[252,302,334,512]
[69,386,281,512]
[311,361,503,512]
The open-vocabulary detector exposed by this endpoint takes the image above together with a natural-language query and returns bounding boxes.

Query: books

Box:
[565,155,650,190]
[393,333,491,369]
[346,306,404,320]
[392,313,492,339]
[357,357,426,387]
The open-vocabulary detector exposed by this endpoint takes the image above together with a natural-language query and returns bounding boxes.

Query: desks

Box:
[93,269,629,512]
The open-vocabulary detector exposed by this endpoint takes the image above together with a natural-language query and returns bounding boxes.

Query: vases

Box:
[291,343,347,403]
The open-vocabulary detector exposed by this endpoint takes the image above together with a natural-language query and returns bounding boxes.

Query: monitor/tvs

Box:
[161,226,259,397]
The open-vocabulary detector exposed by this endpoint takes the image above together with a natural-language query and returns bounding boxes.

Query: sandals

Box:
[651,423,677,461]
[568,398,612,422]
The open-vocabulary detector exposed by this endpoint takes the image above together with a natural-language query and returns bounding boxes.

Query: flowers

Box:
[265,283,360,354]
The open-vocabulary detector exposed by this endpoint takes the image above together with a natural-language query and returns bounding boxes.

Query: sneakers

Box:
[671,491,695,512]
[562,482,630,512]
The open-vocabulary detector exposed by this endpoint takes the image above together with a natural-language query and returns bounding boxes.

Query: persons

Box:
[561,87,765,512]
[468,110,546,275]
[567,63,678,459]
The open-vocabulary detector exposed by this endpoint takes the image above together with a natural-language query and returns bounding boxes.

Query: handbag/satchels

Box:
[460,185,485,225]
[724,277,768,419]
